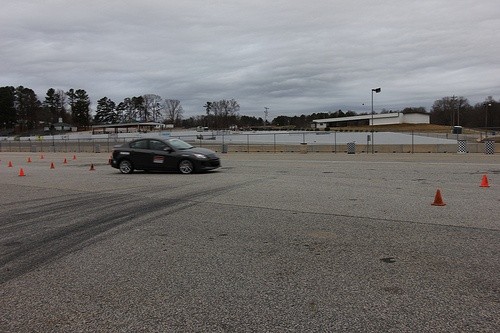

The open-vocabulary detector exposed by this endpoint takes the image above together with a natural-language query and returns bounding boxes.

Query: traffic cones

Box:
[18,168,25,176]
[63,157,68,164]
[430,189,446,206]
[28,157,32,162]
[480,174,489,188]
[49,161,55,169]
[8,161,13,168]
[72,154,77,160]
[90,163,96,170]
[41,154,43,159]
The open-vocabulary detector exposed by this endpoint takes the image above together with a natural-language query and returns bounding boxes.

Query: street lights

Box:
[370,86,383,154]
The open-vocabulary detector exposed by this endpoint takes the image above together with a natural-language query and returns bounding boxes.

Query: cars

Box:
[108,136,220,174]
